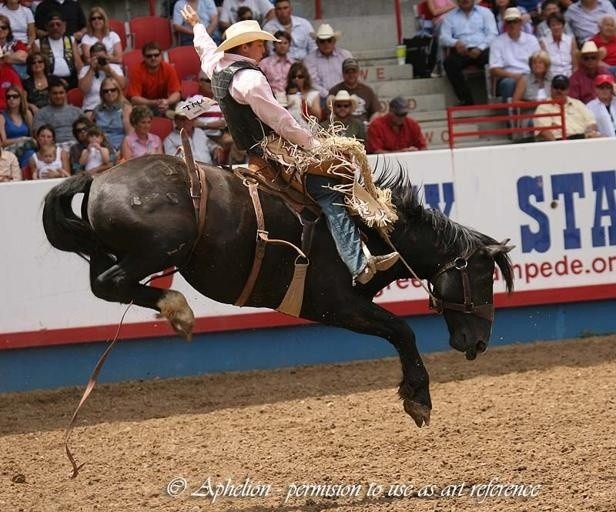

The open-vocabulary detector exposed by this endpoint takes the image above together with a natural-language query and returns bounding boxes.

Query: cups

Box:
[395,44,409,68]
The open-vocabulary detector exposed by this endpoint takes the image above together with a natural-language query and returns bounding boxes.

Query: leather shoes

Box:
[356,252,399,284]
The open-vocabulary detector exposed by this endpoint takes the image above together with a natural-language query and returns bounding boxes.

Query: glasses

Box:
[92,16,101,20]
[76,127,87,133]
[292,74,304,79]
[7,93,19,99]
[146,54,159,58]
[102,88,117,93]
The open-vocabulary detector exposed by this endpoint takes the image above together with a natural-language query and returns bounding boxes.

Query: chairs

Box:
[441,43,485,80]
[485,65,502,103]
[1,15,200,180]
[508,97,536,143]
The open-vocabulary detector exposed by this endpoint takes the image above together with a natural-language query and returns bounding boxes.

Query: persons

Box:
[0,1,616,182]
[180,4,400,287]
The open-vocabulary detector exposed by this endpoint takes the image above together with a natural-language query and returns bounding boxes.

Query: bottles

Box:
[89,141,96,160]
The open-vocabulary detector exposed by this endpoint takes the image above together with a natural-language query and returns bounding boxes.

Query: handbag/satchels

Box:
[403,35,437,79]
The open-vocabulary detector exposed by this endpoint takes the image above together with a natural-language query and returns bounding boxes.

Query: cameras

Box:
[94,57,108,65]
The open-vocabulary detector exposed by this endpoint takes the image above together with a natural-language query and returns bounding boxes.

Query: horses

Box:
[39,153,515,428]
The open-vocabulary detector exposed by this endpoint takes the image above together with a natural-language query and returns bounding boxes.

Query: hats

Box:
[309,24,341,40]
[165,102,202,127]
[343,58,359,73]
[333,91,357,112]
[594,75,613,85]
[503,8,529,24]
[552,75,568,87]
[215,19,281,52]
[389,97,408,114]
[337,104,349,108]
[581,41,606,59]
